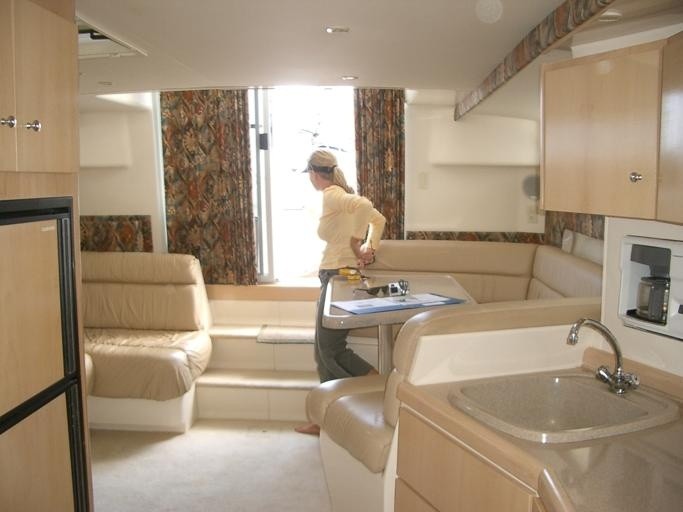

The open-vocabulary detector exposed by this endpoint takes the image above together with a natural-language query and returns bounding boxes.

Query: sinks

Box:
[446,373,681,444]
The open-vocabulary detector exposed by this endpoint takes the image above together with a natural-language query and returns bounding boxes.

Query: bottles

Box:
[637,277,668,324]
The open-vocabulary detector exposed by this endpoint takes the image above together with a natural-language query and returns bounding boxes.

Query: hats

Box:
[300,160,335,175]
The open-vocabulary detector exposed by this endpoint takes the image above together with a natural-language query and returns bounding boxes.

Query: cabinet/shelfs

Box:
[539,28,683,226]
[1,0,83,176]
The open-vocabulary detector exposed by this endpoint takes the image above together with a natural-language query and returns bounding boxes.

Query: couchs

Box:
[308,240,601,511]
[78,250,213,434]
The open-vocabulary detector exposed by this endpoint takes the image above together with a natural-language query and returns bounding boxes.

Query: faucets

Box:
[565,318,640,395]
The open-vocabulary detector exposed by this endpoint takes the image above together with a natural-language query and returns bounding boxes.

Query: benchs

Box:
[198,320,318,422]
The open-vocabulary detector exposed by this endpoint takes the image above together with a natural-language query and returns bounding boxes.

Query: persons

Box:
[294,148,387,433]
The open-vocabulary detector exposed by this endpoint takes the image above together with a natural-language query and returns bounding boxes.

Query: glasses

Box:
[352,283,390,298]
